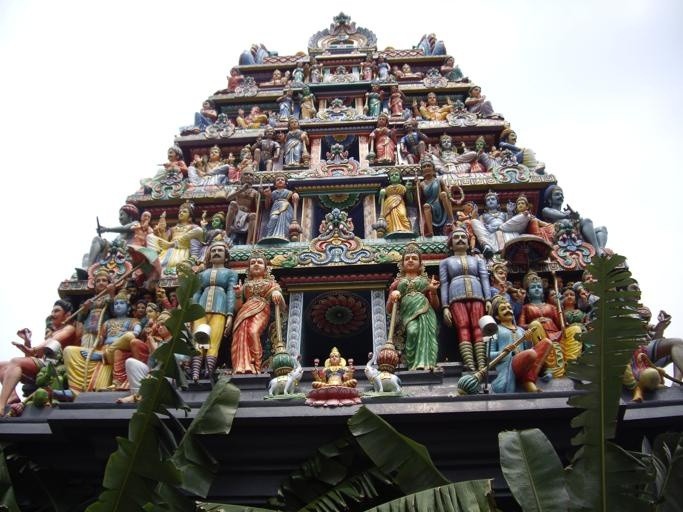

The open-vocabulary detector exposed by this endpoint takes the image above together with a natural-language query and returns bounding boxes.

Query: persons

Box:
[0,241,287,421]
[224,57,470,90]
[311,346,358,388]
[381,229,681,402]
[140,88,315,188]
[374,156,609,259]
[69,174,301,279]
[362,81,546,175]
